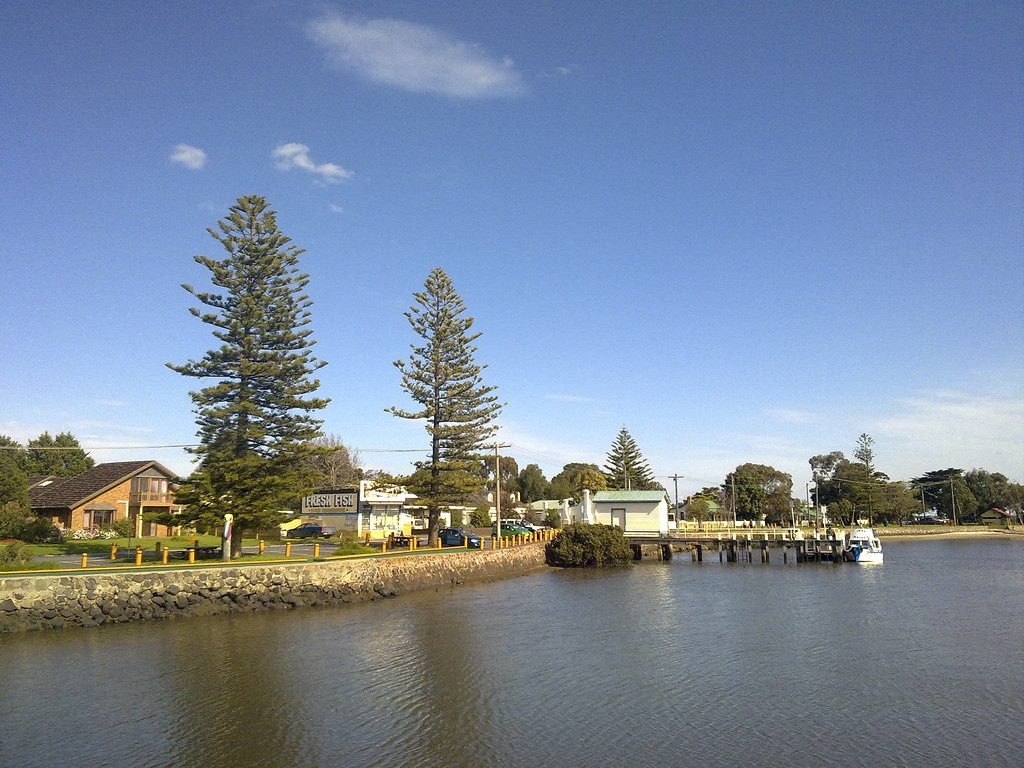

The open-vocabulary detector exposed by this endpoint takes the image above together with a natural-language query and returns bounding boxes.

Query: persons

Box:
[743,519,746,528]
[750,519,752,528]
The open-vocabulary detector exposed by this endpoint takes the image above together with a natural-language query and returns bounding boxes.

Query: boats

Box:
[843,505,884,563]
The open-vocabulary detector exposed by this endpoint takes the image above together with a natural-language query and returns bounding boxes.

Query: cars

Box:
[437,525,486,549]
[492,518,549,542]
[920,516,945,525]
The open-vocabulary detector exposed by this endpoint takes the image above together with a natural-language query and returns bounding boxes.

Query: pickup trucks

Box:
[287,522,337,540]
